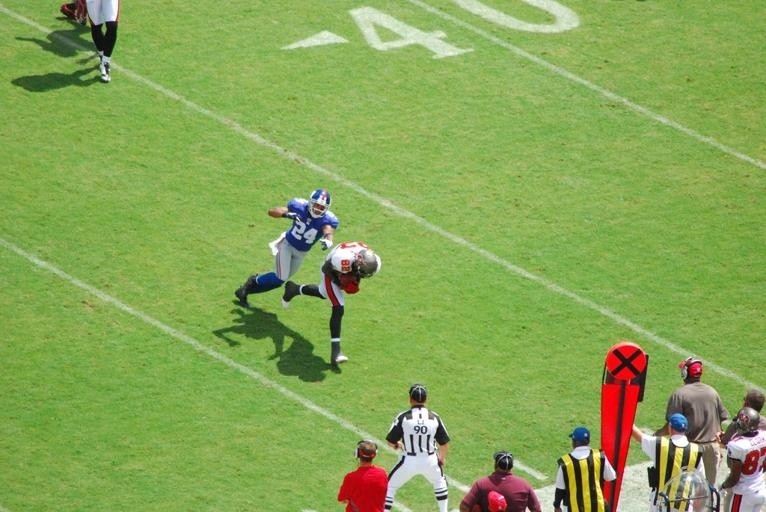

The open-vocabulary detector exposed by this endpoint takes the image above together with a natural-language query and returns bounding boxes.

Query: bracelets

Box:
[281,210,287,218]
[721,482,726,490]
[331,276,341,286]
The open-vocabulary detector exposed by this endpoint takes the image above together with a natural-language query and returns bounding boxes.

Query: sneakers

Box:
[241,274,258,295]
[282,281,295,308]
[331,353,348,365]
[235,287,250,307]
[99,59,111,82]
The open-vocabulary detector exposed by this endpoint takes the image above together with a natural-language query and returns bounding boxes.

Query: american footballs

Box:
[341,272,357,286]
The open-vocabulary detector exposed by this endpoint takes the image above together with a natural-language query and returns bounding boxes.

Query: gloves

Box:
[320,238,333,250]
[287,212,304,223]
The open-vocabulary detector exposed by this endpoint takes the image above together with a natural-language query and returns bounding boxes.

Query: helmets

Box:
[308,189,332,218]
[733,407,760,433]
[357,249,381,278]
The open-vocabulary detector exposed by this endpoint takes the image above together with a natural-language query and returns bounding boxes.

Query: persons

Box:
[714,387,766,450]
[628,413,708,512]
[649,356,733,512]
[233,186,338,308]
[280,240,382,367]
[717,407,766,512]
[84,0,119,83]
[553,426,617,512]
[383,382,451,512]
[459,448,542,512]
[335,439,388,512]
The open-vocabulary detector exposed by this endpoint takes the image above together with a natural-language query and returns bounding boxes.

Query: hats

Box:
[667,412,688,432]
[569,428,590,443]
[488,491,507,512]
[494,451,513,470]
[409,384,427,402]
[359,441,377,458]
[679,357,702,378]
